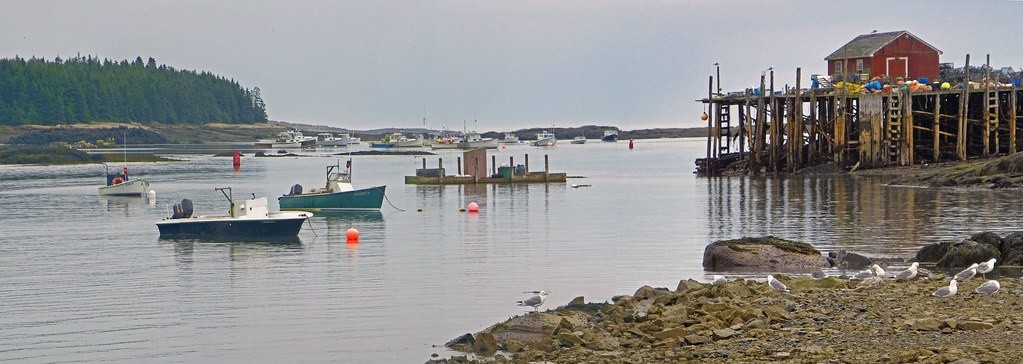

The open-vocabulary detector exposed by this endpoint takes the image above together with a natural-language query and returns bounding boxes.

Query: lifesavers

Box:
[112,177,124,185]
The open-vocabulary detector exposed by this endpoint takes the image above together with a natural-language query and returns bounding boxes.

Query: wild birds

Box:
[709,274,729,286]
[850,264,886,288]
[976,258,996,280]
[516,291,548,316]
[932,280,958,304]
[767,274,790,296]
[975,279,1000,294]
[954,263,980,282]
[889,261,920,287]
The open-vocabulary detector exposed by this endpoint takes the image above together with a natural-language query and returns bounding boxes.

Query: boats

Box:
[98,132,149,197]
[277,158,388,212]
[530,136,557,146]
[599,133,620,142]
[156,187,314,239]
[281,117,520,149]
[571,140,587,144]
